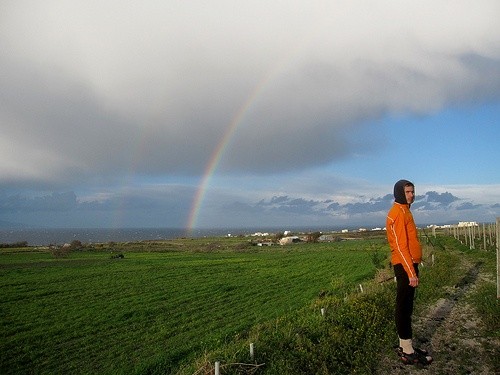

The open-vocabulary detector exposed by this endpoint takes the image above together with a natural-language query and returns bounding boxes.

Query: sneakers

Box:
[397,346,428,356]
[402,351,433,365]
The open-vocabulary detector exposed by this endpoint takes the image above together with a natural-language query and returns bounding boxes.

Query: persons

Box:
[385,178,435,366]
